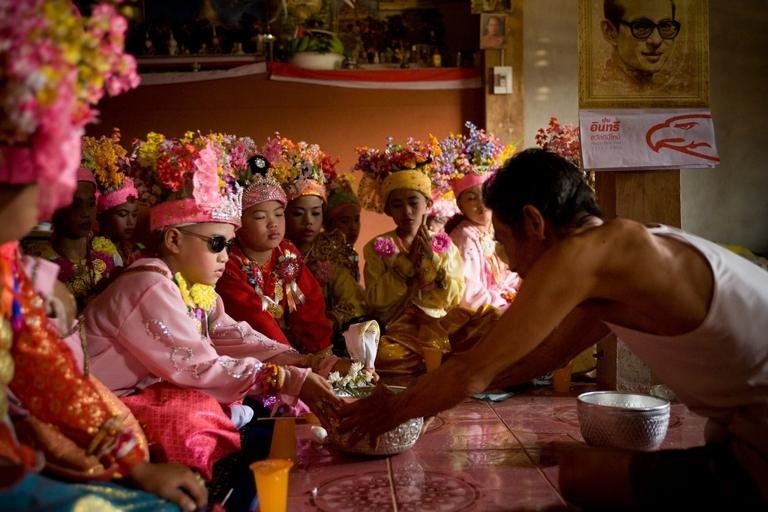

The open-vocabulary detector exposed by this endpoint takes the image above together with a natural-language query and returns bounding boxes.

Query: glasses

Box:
[180,227,236,258]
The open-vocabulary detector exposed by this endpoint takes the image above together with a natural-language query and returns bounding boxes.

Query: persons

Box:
[336,148,768,512]
[594,0,681,97]
[1,1,521,511]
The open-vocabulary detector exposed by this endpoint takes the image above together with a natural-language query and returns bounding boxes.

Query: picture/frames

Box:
[480,13,508,50]
[579,1,710,109]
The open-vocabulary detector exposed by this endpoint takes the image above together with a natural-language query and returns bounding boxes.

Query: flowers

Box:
[373,237,396,255]
[328,360,374,399]
[431,232,451,252]
[277,251,301,282]
[238,257,266,285]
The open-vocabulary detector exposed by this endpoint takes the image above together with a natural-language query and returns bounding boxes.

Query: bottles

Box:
[431,48,442,68]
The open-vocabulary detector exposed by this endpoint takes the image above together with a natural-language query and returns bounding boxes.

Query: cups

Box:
[554,361,573,394]
[419,346,440,377]
[266,420,302,467]
[249,460,292,512]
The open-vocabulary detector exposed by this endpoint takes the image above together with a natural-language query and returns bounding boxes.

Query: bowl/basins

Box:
[284,52,348,72]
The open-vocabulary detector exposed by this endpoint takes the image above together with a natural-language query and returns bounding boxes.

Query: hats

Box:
[99,133,137,207]
[143,129,242,231]
[329,170,362,218]
[78,159,96,187]
[287,140,327,205]
[2,1,84,184]
[440,121,517,197]
[358,140,432,201]
[243,135,287,209]
